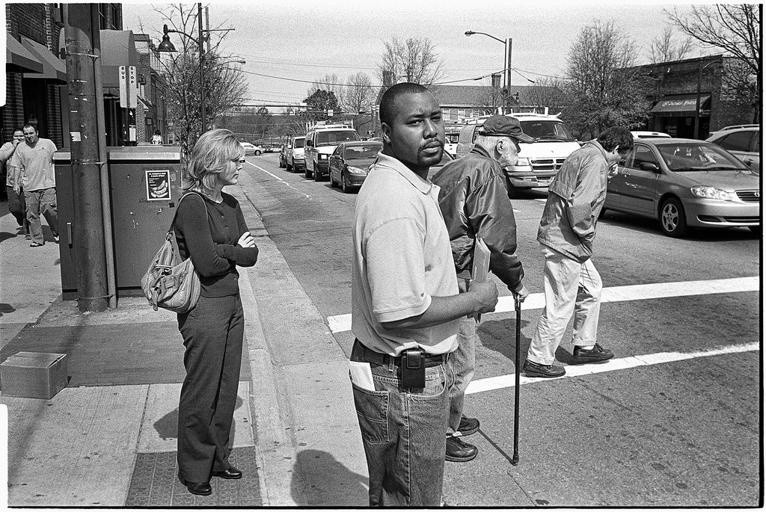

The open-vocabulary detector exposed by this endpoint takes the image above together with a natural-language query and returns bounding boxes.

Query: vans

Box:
[457,112,582,195]
[303,120,363,182]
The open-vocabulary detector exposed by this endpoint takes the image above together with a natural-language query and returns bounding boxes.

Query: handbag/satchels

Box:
[141,230,201,315]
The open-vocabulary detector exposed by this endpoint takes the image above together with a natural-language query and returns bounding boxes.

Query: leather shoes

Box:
[212,466,242,479]
[178,472,211,495]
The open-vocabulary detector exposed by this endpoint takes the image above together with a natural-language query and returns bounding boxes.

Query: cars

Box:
[278,145,287,169]
[602,139,760,237]
[329,141,383,193]
[427,151,456,181]
[631,131,672,140]
[444,133,459,156]
[240,142,264,156]
[264,144,282,153]
[366,137,384,142]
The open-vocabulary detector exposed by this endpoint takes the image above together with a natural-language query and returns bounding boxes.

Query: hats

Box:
[478,114,536,143]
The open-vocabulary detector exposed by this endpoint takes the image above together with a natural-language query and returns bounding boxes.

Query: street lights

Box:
[465,30,513,113]
[202,60,247,136]
[154,24,205,134]
[694,55,725,140]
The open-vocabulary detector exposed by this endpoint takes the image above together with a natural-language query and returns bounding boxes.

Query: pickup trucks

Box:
[284,136,306,172]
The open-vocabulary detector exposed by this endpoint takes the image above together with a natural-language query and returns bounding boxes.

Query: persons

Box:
[429,116,533,462]
[523,126,634,377]
[348,83,499,509]
[10,123,60,247]
[168,129,258,496]
[149,130,164,144]
[0,128,31,240]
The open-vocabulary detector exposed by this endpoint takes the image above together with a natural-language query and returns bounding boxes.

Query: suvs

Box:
[705,124,761,173]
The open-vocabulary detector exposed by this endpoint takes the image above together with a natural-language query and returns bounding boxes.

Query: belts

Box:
[383,353,442,368]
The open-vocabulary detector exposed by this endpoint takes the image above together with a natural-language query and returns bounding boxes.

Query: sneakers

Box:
[523,358,565,377]
[446,436,478,462]
[573,342,613,362]
[457,417,480,435]
